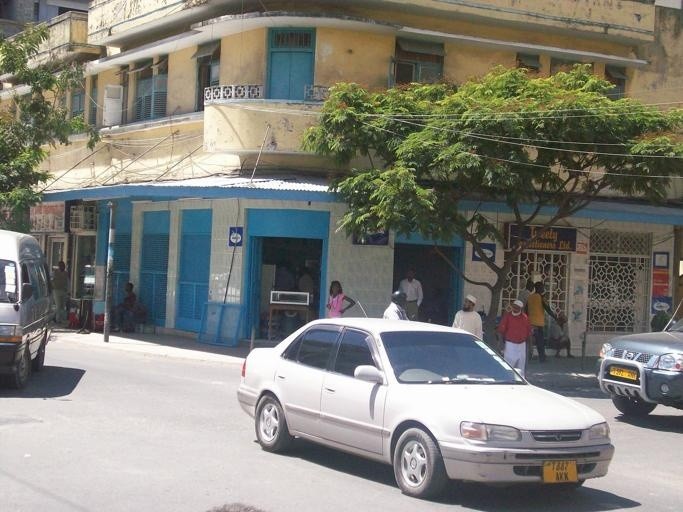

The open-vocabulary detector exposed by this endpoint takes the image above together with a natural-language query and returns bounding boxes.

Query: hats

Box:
[465,294,477,305]
[654,302,670,311]
[513,301,523,308]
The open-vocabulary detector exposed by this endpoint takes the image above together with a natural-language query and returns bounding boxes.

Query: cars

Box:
[236,315,615,498]
[83,264,96,292]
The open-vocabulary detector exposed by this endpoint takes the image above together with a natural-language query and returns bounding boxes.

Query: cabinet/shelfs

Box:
[266,304,309,341]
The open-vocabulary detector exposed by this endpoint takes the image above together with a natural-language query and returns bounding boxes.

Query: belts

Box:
[505,339,525,343]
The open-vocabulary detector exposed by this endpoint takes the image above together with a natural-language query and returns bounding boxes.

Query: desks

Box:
[69,295,103,335]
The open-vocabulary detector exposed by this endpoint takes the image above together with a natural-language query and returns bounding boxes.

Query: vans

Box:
[0,229,56,390]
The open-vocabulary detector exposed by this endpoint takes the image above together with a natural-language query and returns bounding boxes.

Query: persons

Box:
[278,265,576,380]
[53,261,147,333]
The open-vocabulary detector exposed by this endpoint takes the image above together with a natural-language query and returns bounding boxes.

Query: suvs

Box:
[594,318,683,417]
